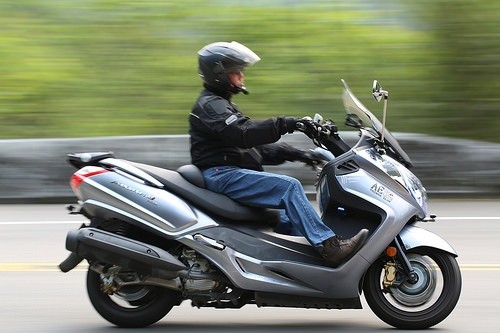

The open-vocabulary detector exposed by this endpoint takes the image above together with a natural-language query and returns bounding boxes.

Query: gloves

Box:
[285,116,329,141]
[301,152,323,168]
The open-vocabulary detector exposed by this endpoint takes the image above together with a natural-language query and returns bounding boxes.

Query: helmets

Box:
[196,41,260,95]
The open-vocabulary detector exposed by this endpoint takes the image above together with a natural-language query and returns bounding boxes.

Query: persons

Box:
[187,41,369,268]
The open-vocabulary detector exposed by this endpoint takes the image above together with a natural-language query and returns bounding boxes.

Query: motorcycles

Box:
[60,77,462,329]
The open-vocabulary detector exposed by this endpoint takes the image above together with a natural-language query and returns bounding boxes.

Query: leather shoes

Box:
[321,229,369,268]
